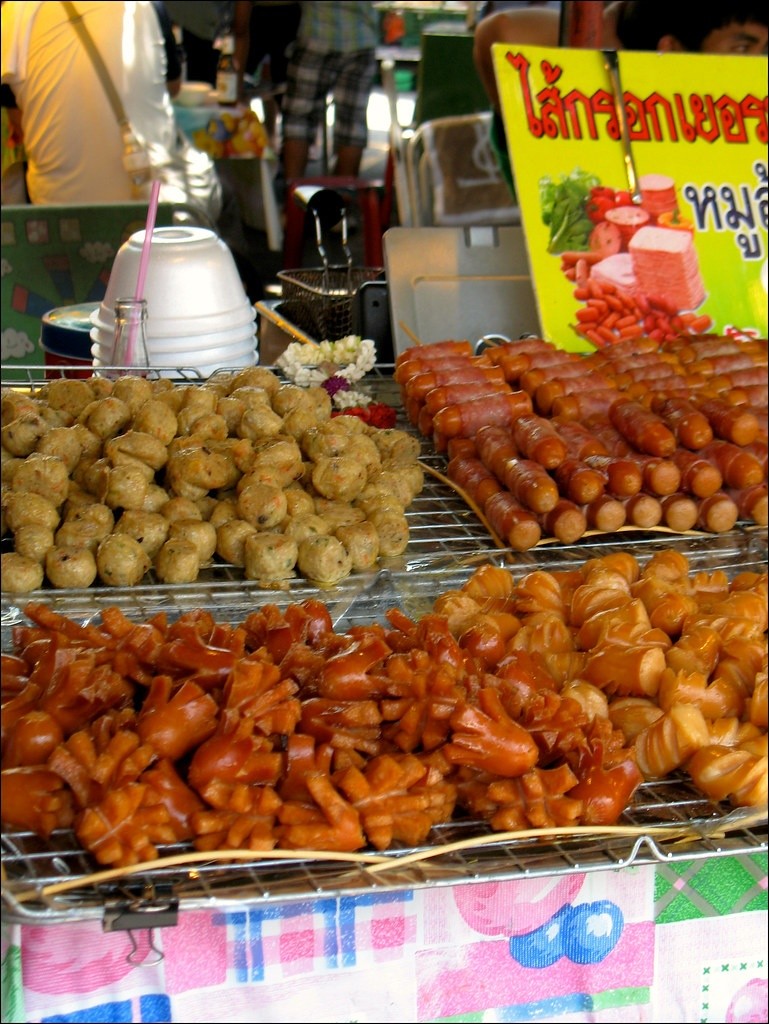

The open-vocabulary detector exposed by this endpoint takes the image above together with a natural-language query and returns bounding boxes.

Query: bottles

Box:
[217,25,238,107]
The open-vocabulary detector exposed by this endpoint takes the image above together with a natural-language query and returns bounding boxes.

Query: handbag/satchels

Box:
[123,128,225,233]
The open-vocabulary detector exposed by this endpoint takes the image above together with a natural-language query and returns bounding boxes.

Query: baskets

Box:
[274,263,385,342]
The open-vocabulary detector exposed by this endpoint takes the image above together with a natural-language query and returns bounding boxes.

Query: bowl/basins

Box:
[90,228,260,377]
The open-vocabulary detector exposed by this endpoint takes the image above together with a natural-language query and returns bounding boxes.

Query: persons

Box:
[1,1,194,207]
[473,0,769,212]
[278,0,381,234]
[218,0,304,160]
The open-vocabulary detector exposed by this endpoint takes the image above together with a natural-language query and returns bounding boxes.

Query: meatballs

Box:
[2,346,425,586]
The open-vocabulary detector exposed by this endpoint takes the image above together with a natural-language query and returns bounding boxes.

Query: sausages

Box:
[401,309,769,606]
[2,606,767,895]
